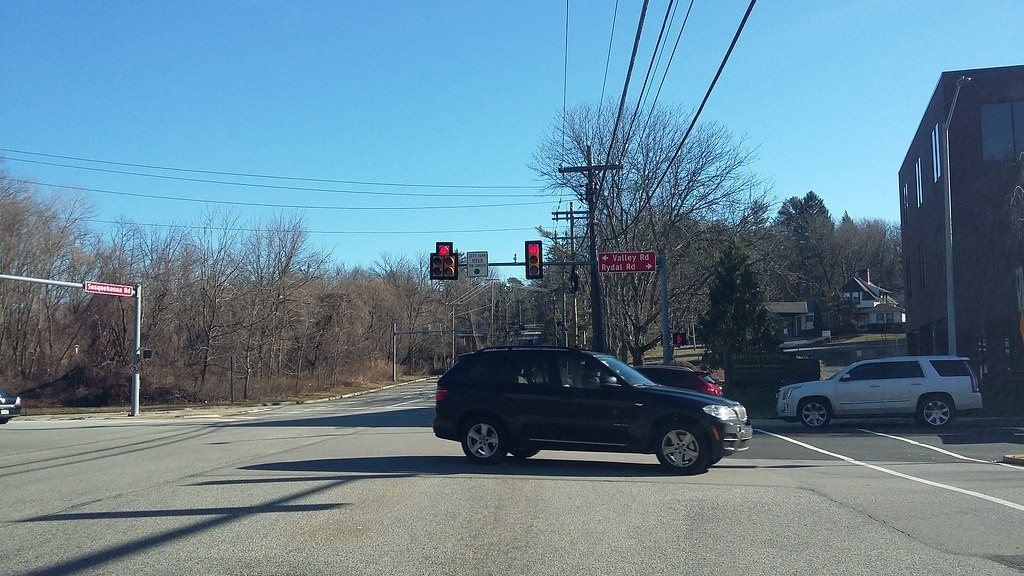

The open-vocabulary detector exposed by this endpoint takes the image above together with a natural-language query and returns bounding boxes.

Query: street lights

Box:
[942,76,972,355]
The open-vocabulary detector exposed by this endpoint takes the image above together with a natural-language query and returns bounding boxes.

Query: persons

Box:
[582,363,601,391]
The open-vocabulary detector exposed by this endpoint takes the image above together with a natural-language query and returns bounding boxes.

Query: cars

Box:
[0,389,21,424]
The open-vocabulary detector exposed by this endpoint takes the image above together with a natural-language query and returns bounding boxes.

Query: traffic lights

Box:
[137,348,152,361]
[525,240,544,279]
[670,331,686,347]
[430,242,458,280]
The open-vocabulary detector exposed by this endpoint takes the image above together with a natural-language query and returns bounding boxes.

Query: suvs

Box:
[629,365,723,399]
[433,345,753,475]
[775,355,983,431]
